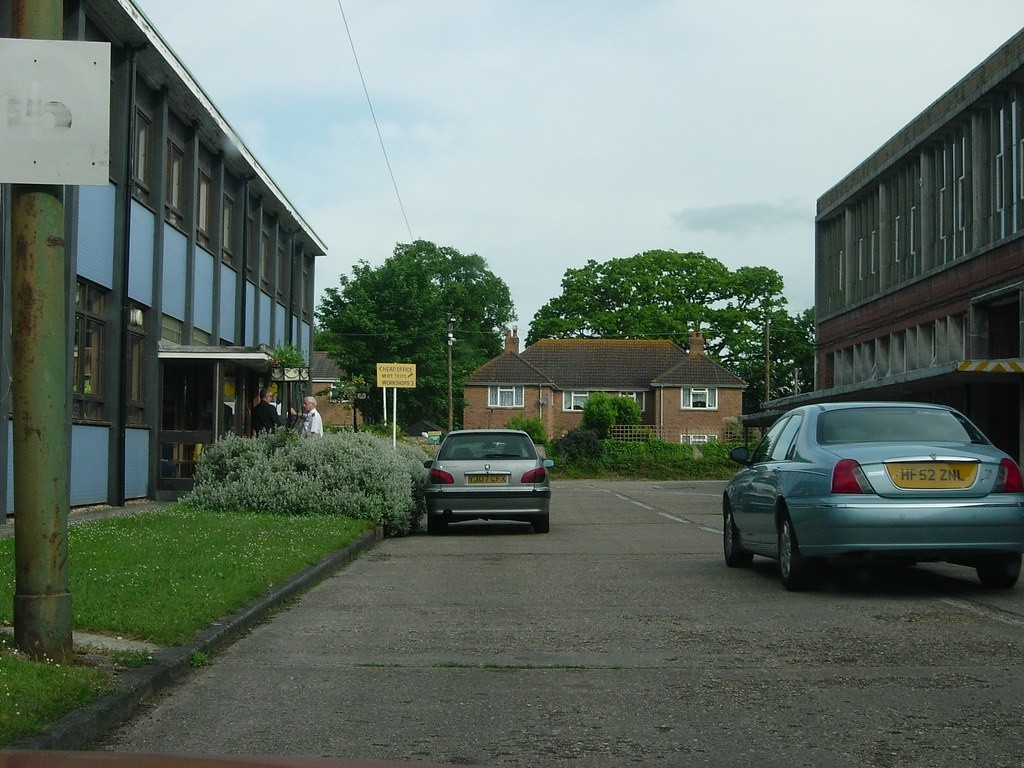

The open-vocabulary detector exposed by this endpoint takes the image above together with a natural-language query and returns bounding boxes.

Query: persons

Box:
[250,386,281,435]
[288,395,322,438]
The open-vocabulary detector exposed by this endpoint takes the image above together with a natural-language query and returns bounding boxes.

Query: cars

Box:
[423,429,554,534]
[720,401,1024,594]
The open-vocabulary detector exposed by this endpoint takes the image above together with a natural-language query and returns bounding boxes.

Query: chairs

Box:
[503,443,522,457]
[453,447,474,459]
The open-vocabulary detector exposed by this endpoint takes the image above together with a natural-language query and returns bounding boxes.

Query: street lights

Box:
[445,312,458,432]
